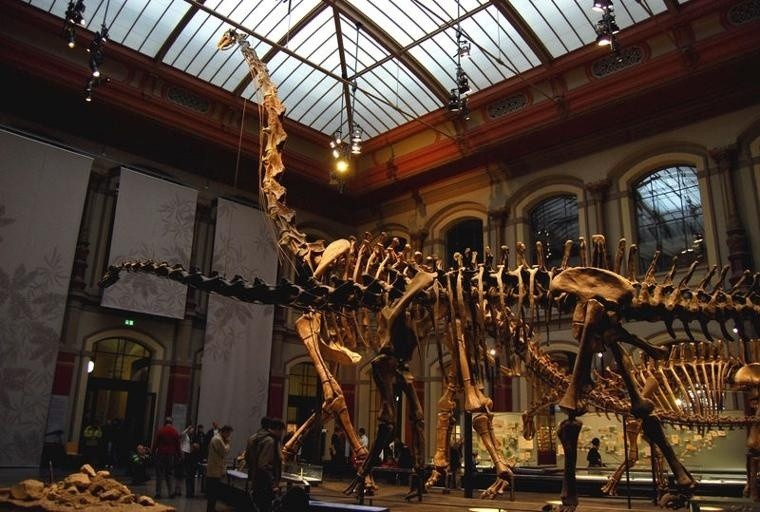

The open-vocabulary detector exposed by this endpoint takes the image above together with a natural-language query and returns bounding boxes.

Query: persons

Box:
[587,438,606,475]
[245,418,287,512]
[78,412,233,512]
[257,416,270,434]
[359,428,368,448]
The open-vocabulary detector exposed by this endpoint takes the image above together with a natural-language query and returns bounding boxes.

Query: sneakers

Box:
[153,492,211,500]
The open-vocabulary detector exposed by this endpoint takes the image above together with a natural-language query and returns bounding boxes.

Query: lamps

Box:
[448,0,476,129]
[60,0,111,102]
[326,21,364,193]
[591,0,623,61]
